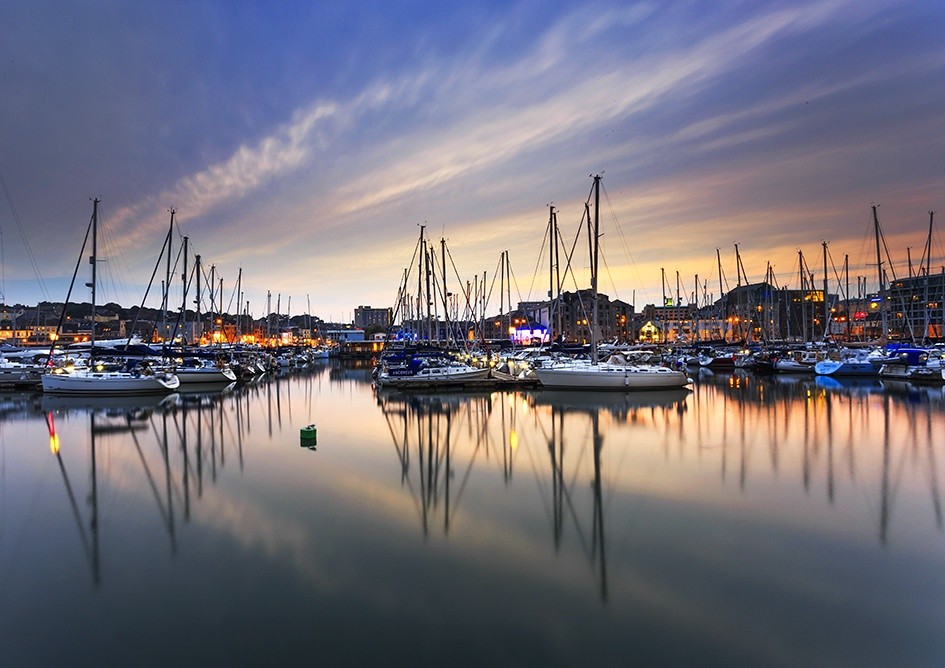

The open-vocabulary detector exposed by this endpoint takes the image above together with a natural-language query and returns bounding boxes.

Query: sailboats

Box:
[1,189,342,399]
[370,166,945,394]
